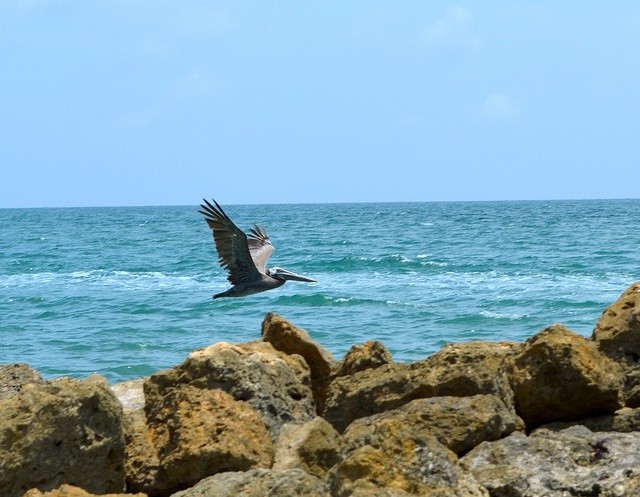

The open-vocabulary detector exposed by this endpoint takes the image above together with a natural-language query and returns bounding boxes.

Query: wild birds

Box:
[197,196,317,299]
